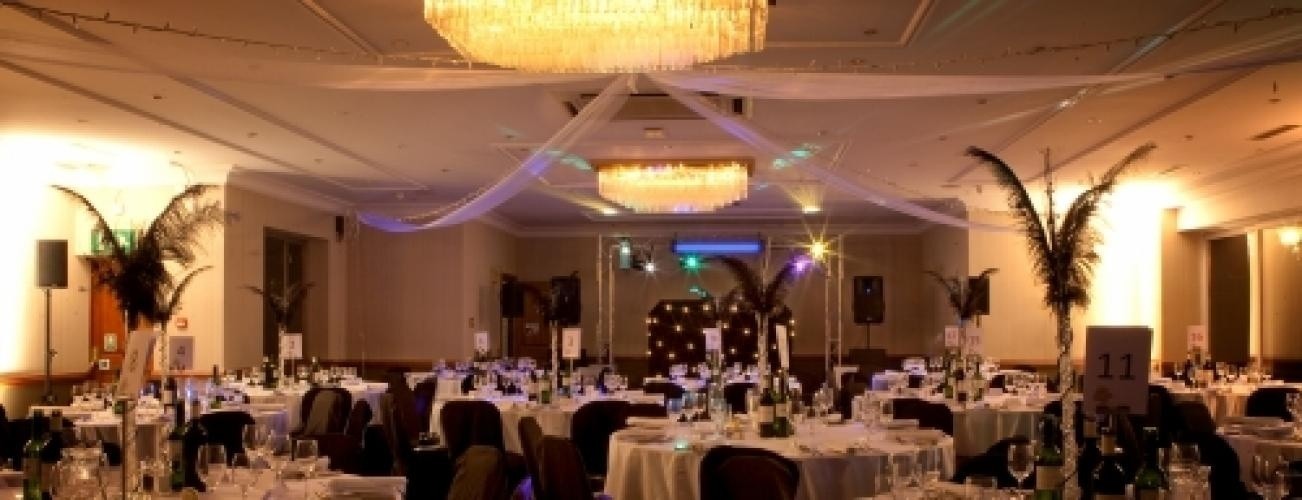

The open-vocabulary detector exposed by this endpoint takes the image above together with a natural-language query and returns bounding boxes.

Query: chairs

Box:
[289,389,353,459]
[699,445,800,500]
[340,425,395,478]
[397,381,437,444]
[884,398,952,433]
[445,444,498,500]
[198,411,254,467]
[725,383,758,414]
[5,418,77,471]
[440,402,502,447]
[571,401,629,476]
[518,418,544,461]
[531,434,593,500]
[643,383,685,398]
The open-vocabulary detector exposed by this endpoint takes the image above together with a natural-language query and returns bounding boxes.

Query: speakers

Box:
[852,273,885,326]
[966,275,991,316]
[551,276,581,324]
[500,281,526,319]
[36,238,71,290]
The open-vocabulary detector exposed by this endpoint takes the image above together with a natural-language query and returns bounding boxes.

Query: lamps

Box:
[587,156,756,213]
[424,0,767,76]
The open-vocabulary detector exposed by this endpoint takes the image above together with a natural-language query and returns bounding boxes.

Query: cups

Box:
[1264,463,1292,500]
[268,434,291,492]
[888,453,914,498]
[683,393,696,421]
[668,399,681,426]
[196,444,226,496]
[1253,454,1283,499]
[1172,444,1198,480]
[243,425,267,476]
[880,397,893,423]
[1008,444,1035,491]
[980,488,1011,500]
[914,446,940,495]
[1174,361,1182,381]
[695,392,707,418]
[231,453,259,499]
[965,476,998,500]
[295,438,317,500]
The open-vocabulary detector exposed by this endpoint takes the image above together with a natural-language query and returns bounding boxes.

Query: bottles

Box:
[163,376,177,416]
[541,368,551,404]
[1093,428,1126,500]
[1134,427,1169,500]
[759,374,774,438]
[38,410,67,500]
[528,365,537,404]
[945,360,953,398]
[182,399,208,492]
[1185,352,1194,388]
[23,409,46,500]
[260,354,270,385]
[1035,415,1064,500]
[168,400,188,491]
[310,353,320,386]
[776,375,790,438]
[211,365,222,409]
[973,362,983,401]
